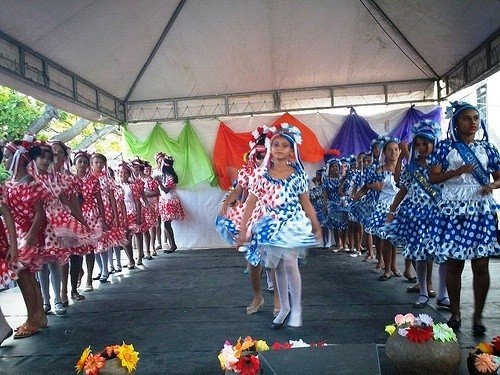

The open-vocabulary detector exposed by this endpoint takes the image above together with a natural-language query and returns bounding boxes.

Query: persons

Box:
[213,99,500,331]
[1,134,188,347]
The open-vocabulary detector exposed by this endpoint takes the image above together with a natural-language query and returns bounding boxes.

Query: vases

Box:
[98,357,128,375]
[468,349,500,375]
[386,323,461,375]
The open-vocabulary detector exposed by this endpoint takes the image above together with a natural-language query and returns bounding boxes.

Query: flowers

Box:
[385,313,458,344]
[76,340,140,375]
[217,336,331,375]
[474,335,500,375]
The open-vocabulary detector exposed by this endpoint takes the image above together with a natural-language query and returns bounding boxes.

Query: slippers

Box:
[13,322,48,338]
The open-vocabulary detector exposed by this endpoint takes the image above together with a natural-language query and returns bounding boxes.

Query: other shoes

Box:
[246,298,266,314]
[286,318,303,332]
[267,282,274,293]
[274,310,279,317]
[404,271,418,283]
[407,287,420,293]
[244,269,249,273]
[428,288,436,297]
[325,242,402,281]
[448,313,463,328]
[0,327,13,345]
[61,293,69,306]
[77,238,176,293]
[473,316,485,334]
[55,299,66,314]
[70,291,86,300]
[413,295,428,308]
[43,304,51,313]
[271,310,291,330]
[437,297,451,308]
